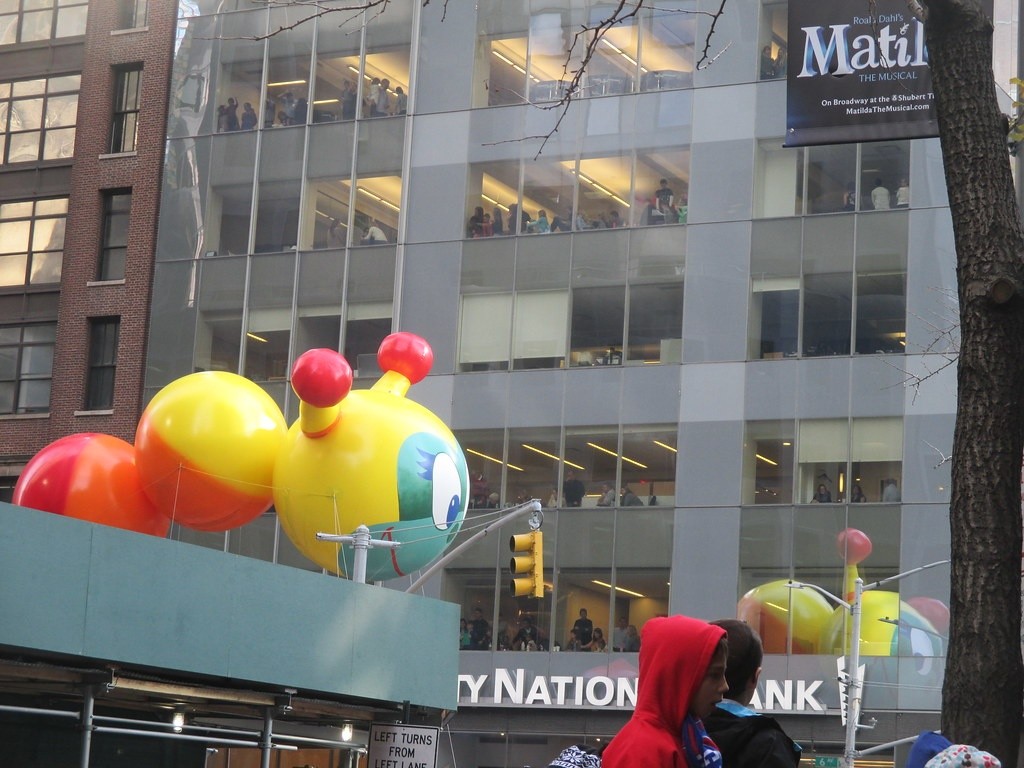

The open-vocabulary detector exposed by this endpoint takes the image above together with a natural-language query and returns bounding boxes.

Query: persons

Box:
[575,178,690,231]
[762,45,787,78]
[576,346,622,366]
[810,484,832,502]
[340,77,407,119]
[883,478,901,502]
[599,613,730,768]
[264,90,308,129]
[701,617,803,768]
[326,218,348,249]
[852,485,866,503]
[842,176,909,210]
[362,227,389,245]
[468,203,572,236]
[216,97,258,132]
[459,607,643,652]
[469,468,645,506]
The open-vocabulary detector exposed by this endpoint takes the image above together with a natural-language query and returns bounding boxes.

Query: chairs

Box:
[649,496,657,506]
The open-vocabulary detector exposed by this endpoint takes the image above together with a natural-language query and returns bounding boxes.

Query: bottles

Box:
[521,641,525,650]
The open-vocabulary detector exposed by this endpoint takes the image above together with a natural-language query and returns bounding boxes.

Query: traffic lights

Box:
[510,530,544,601]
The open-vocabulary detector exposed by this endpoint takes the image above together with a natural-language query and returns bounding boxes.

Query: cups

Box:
[553,646,560,651]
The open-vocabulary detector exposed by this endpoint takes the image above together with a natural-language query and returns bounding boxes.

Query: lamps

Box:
[348,65,401,96]
[307,98,339,105]
[601,37,648,73]
[572,169,631,209]
[267,79,306,87]
[315,195,349,228]
[491,50,541,83]
[358,187,400,212]
[481,191,510,213]
[340,719,353,741]
[171,703,185,733]
